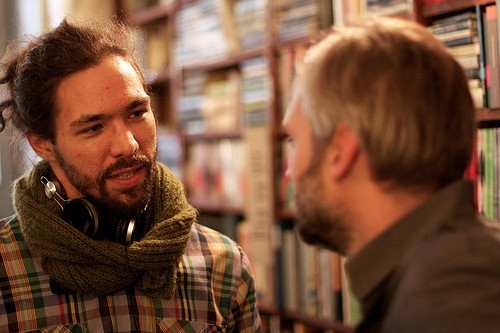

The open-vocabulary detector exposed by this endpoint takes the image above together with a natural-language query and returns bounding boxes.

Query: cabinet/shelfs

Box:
[110,0,500,333]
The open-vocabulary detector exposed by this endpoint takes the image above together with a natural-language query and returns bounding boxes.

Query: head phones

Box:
[40,175,154,245]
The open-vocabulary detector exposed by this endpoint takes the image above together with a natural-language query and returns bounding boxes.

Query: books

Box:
[424,14,500,222]
[278,140,365,327]
[279,0,405,87]
[133,0,269,304]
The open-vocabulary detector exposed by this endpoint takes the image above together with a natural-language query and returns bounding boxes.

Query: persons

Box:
[280,12,500,332]
[0,18,266,333]
